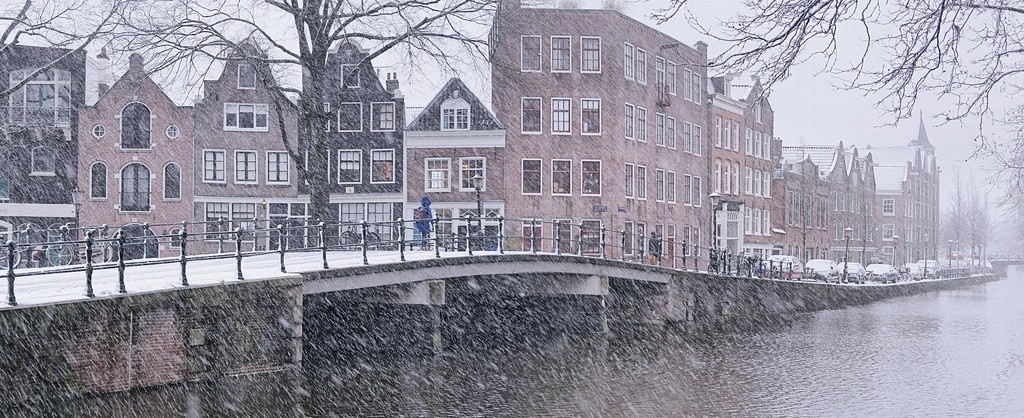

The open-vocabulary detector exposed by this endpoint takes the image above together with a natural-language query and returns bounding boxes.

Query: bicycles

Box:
[0,233,22,270]
[46,221,113,267]
[338,220,383,252]
[707,248,785,279]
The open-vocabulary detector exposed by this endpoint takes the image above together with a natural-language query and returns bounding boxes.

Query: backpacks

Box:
[414,207,424,223]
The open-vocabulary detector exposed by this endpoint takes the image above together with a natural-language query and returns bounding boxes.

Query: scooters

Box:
[894,266,914,282]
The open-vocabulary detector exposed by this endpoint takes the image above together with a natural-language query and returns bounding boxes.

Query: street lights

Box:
[842,227,853,284]
[891,235,900,267]
[709,191,721,259]
[472,172,482,252]
[922,233,930,279]
[947,239,954,269]
[72,186,81,242]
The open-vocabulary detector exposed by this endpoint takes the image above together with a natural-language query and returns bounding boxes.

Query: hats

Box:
[651,232,655,235]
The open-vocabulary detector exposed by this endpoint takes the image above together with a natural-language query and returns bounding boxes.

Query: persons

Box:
[32,245,51,268]
[414,197,435,251]
[648,230,663,265]
[705,243,762,280]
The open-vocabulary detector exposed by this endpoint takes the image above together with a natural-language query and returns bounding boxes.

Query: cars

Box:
[838,262,866,284]
[939,258,993,277]
[866,264,898,284]
[902,263,923,282]
[917,259,941,280]
[758,255,804,280]
[802,258,841,284]
